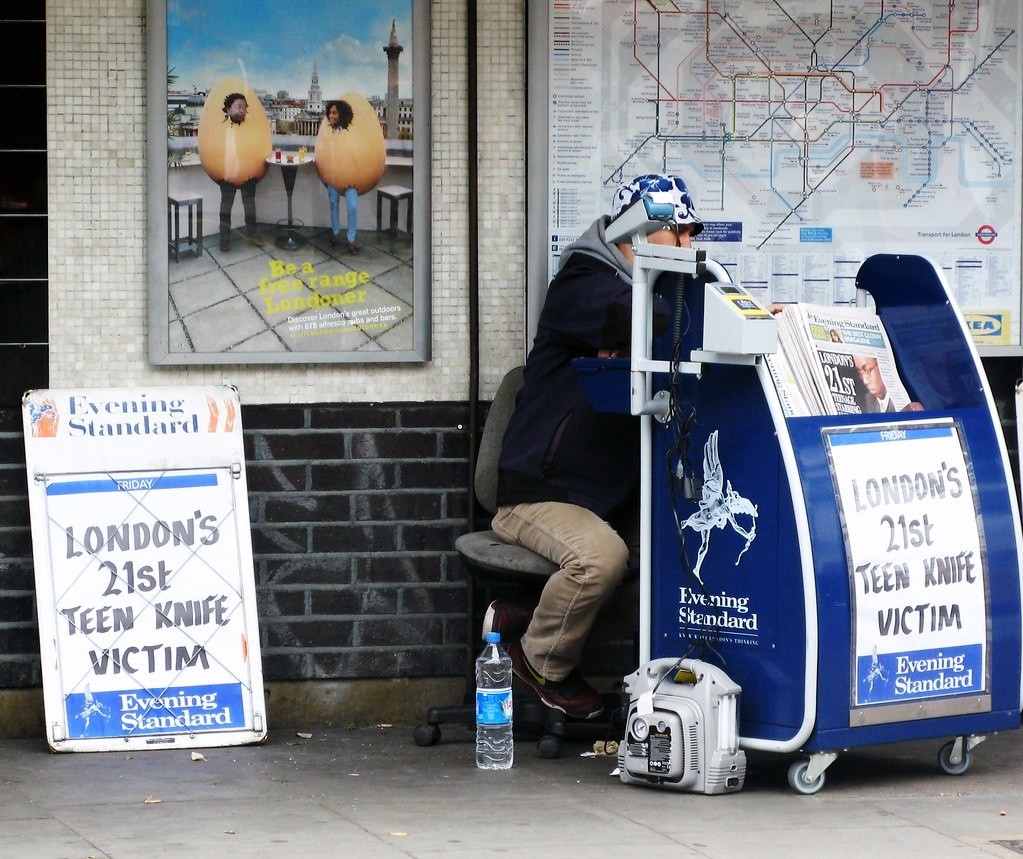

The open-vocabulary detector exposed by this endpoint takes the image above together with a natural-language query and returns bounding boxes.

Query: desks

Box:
[265,156,313,250]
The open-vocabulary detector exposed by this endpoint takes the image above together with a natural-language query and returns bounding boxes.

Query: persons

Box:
[493,173,788,721]
[220,94,265,252]
[830,329,842,343]
[326,101,360,255]
[852,355,896,413]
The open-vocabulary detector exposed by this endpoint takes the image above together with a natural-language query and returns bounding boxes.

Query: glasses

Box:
[857,364,877,379]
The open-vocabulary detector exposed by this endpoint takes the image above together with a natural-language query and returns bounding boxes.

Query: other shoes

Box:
[331,235,342,247]
[220,236,231,251]
[348,244,358,255]
[250,235,263,246]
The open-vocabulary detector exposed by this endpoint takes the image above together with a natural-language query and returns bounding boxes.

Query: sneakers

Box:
[482,586,541,641]
[507,643,605,720]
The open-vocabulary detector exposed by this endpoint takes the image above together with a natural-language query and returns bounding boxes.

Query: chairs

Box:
[415,365,626,758]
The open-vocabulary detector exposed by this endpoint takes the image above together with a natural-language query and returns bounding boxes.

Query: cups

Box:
[298,147,307,162]
[285,152,294,161]
[275,147,282,163]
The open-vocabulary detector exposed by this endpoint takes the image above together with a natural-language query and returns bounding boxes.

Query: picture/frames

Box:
[147,0,432,366]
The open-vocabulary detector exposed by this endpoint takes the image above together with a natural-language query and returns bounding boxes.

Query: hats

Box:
[610,176,704,235]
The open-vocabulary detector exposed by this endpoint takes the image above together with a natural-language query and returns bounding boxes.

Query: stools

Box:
[167,193,203,263]
[377,185,413,252]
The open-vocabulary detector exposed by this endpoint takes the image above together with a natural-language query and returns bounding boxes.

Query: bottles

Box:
[475,632,515,769]
